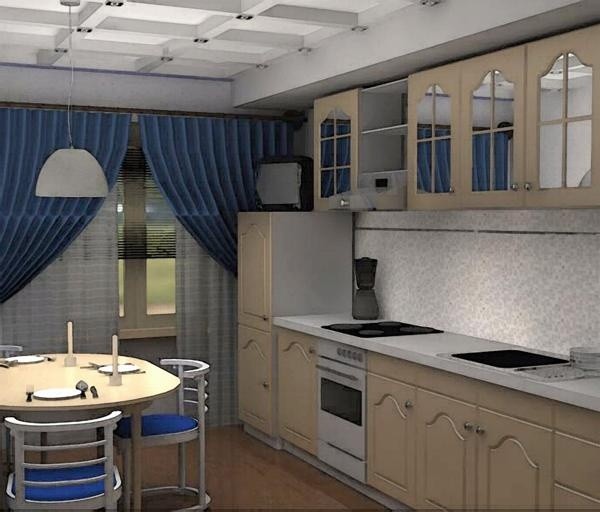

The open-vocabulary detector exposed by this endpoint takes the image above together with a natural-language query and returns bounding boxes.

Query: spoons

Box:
[76,379,88,399]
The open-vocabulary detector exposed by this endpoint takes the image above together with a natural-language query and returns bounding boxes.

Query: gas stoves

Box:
[321,321,445,338]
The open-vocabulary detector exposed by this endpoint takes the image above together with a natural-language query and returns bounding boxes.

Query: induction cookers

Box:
[436,348,586,381]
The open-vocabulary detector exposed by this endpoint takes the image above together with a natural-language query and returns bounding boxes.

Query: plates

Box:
[98,364,141,374]
[33,387,81,399]
[6,355,45,364]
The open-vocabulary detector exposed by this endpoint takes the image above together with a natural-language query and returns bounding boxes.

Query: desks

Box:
[1,350,181,511]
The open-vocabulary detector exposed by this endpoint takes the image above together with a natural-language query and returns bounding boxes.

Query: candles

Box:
[66,319,74,356]
[110,334,119,376]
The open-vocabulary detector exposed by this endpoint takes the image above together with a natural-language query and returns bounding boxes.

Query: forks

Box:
[25,384,34,402]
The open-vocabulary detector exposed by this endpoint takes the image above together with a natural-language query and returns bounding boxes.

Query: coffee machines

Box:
[352,256,379,319]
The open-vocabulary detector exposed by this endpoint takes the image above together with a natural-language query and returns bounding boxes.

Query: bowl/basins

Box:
[570,347,600,371]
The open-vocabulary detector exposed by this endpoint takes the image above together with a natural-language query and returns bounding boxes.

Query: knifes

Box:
[90,386,98,398]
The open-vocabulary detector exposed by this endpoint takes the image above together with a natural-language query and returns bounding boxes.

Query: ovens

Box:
[315,338,368,486]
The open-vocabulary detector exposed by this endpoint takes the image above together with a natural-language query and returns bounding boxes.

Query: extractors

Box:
[327,169,407,211]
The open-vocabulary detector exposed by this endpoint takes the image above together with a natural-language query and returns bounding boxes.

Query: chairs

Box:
[1,343,48,473]
[4,409,125,512]
[104,358,215,512]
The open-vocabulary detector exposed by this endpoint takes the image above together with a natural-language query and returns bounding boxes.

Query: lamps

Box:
[35,0,111,200]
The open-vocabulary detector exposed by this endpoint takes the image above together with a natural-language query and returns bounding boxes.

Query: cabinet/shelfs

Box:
[313,79,407,210]
[276,325,319,458]
[364,350,418,511]
[460,22,600,210]
[407,59,460,210]
[554,401,600,510]
[419,365,555,511]
